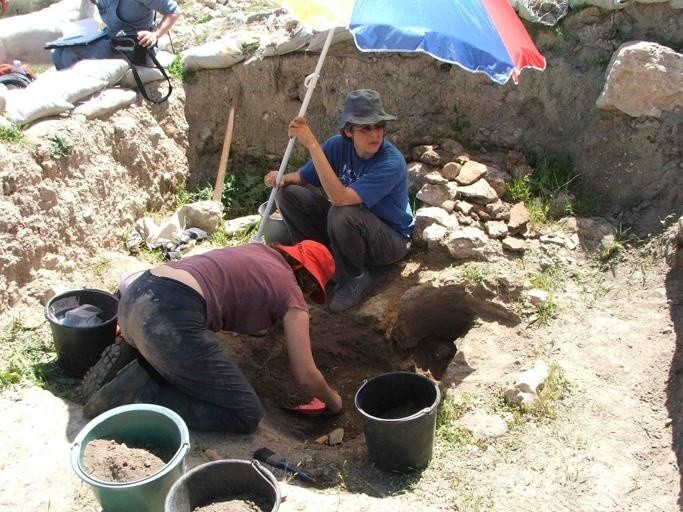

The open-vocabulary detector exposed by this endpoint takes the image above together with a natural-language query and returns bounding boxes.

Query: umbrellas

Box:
[256,0,546,240]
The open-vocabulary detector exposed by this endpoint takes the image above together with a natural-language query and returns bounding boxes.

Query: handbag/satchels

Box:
[43,28,121,69]
[111,34,147,67]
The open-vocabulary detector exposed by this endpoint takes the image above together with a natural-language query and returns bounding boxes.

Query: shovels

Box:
[316,427,344,445]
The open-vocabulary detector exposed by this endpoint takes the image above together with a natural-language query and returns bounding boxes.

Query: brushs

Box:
[253,447,317,483]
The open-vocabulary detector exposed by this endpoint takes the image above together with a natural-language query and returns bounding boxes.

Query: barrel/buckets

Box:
[164,459,281,512]
[257,200,291,246]
[44,289,119,377]
[353,371,441,474]
[69,402,190,512]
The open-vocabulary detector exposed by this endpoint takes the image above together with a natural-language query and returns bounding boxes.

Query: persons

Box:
[263,89,415,313]
[78,240,342,433]
[51,0,180,71]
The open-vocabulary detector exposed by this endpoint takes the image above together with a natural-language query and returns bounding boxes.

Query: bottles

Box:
[10,59,27,77]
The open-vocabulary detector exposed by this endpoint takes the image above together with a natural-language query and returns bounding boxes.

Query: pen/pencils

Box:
[63,35,82,40]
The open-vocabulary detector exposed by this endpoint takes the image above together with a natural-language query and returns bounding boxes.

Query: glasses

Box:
[352,123,385,133]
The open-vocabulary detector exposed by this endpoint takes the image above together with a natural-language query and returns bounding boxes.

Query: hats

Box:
[274,238,336,304]
[334,89,398,130]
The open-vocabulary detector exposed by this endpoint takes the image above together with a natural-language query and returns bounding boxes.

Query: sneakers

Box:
[328,268,374,314]
[80,359,152,418]
[80,343,130,405]
